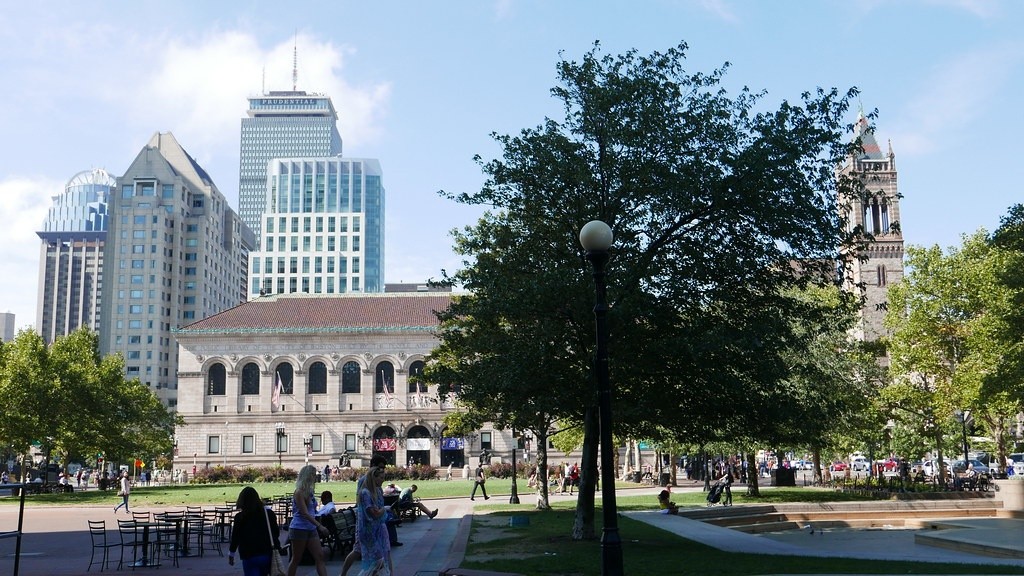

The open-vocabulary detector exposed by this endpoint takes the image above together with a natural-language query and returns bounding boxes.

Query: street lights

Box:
[275,422,285,466]
[578,219,625,576]
[302,433,313,467]
[954,408,972,471]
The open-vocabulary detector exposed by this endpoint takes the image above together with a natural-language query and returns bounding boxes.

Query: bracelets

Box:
[314,521,321,526]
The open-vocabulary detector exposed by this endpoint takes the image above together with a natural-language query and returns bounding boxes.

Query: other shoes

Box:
[391,541,403,546]
[485,496,489,500]
[470,498,474,501]
[597,489,599,491]
[430,509,438,519]
[570,493,572,495]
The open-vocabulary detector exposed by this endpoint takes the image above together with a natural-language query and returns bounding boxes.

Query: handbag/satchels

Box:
[476,476,485,484]
[270,549,286,576]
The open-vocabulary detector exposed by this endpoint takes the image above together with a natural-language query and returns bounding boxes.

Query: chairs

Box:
[87,492,422,574]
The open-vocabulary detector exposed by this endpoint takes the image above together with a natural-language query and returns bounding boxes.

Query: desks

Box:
[120,522,171,567]
[155,517,210,559]
[211,510,232,544]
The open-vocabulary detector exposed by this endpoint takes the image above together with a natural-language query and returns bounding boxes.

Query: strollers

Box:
[706,481,727,508]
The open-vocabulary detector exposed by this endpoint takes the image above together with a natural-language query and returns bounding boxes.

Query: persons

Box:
[408,456,416,465]
[445,463,452,481]
[480,448,491,465]
[153,468,181,486]
[0,471,10,484]
[324,465,330,482]
[75,467,100,491]
[282,491,336,556]
[879,458,975,478]
[113,470,131,515]
[471,455,796,507]
[59,474,74,493]
[102,467,124,480]
[146,469,151,486]
[386,513,403,546]
[229,485,282,576]
[288,466,330,576]
[339,454,395,576]
[140,469,146,486]
[399,483,438,519]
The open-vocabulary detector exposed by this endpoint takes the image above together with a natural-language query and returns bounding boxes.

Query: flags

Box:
[272,376,422,408]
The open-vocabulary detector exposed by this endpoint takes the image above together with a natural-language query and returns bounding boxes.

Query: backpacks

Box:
[568,465,574,475]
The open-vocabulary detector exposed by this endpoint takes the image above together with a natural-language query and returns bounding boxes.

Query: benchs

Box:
[909,472,989,492]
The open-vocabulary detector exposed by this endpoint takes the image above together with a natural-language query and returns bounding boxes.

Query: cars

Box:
[783,451,1024,479]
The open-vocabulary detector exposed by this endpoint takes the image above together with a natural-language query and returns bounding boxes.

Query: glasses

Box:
[374,468,380,474]
